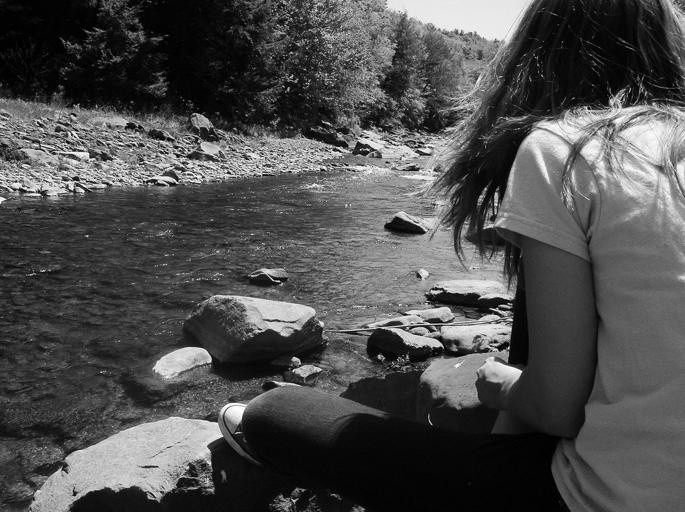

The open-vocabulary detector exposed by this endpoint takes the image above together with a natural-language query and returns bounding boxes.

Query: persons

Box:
[217,0,685,512]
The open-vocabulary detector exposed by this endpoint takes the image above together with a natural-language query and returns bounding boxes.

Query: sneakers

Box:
[218,402,263,468]
